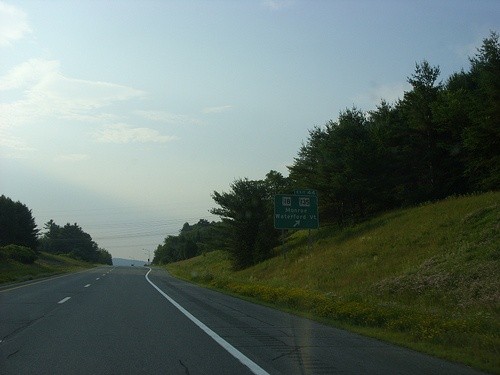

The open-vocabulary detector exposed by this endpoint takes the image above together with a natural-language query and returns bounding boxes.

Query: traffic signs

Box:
[273,189,319,230]
